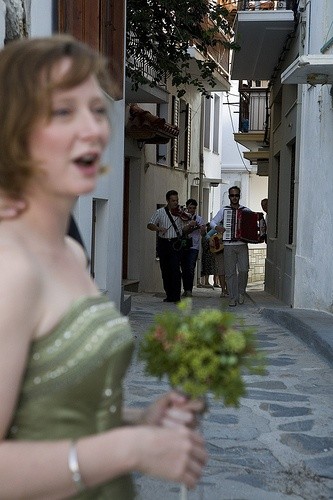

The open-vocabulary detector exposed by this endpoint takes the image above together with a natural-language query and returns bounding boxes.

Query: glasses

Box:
[230,194,240,197]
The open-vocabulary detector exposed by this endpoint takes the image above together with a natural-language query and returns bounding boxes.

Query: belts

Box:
[158,237,180,241]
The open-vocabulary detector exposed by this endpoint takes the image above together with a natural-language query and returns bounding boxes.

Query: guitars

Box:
[208,234,225,253]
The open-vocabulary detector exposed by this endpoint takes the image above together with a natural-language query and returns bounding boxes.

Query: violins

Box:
[171,204,202,230]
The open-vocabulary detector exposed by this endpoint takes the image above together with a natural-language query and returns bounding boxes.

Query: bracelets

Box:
[68,439,88,494]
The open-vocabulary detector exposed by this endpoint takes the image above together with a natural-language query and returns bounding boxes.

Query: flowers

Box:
[135,295,263,500]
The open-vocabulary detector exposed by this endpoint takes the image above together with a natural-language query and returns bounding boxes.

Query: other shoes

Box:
[182,291,192,297]
[204,282,212,288]
[214,284,220,287]
[163,296,180,303]
[238,293,245,304]
[225,292,230,297]
[229,296,235,306]
[220,291,224,297]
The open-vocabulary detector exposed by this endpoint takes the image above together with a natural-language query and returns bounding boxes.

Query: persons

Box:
[181,200,230,298]
[148,190,193,302]
[210,186,265,307]
[0,37,207,500]
[261,199,267,245]
